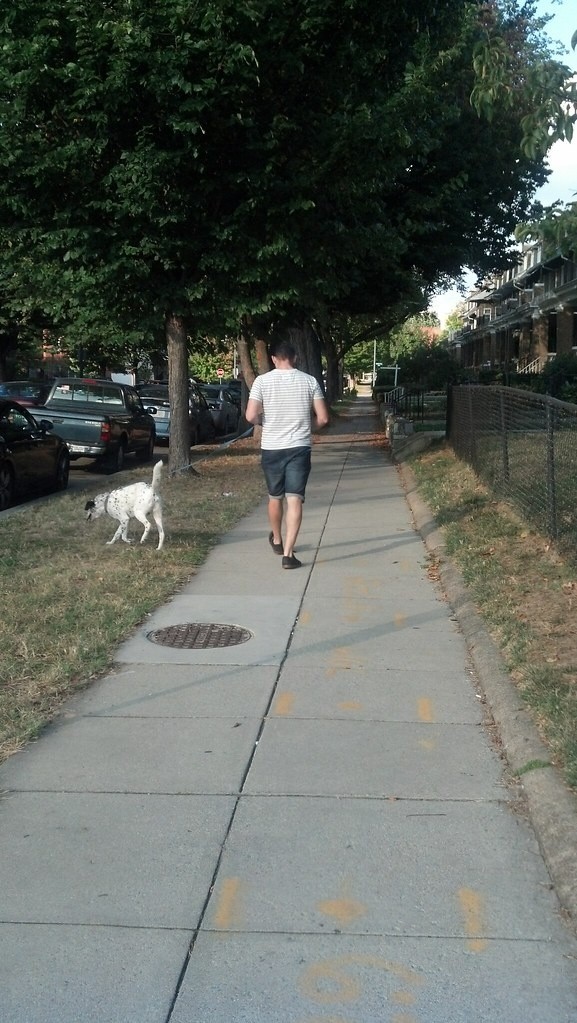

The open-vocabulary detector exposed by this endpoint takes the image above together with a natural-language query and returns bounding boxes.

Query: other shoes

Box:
[269,531,283,555]
[282,551,301,569]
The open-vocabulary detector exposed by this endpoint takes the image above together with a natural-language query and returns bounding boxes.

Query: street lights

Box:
[221,340,236,379]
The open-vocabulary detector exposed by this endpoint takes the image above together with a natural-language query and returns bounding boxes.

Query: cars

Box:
[134,381,216,446]
[0,399,69,510]
[0,381,56,408]
[190,379,242,435]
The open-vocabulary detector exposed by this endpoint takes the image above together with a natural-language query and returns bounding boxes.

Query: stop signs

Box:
[217,369,224,375]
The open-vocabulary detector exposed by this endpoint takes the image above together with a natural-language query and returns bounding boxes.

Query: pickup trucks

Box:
[9,377,158,474]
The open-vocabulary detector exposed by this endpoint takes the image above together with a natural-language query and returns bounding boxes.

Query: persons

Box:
[245,339,329,569]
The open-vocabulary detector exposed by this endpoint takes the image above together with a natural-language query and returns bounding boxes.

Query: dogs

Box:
[86,460,167,551]
